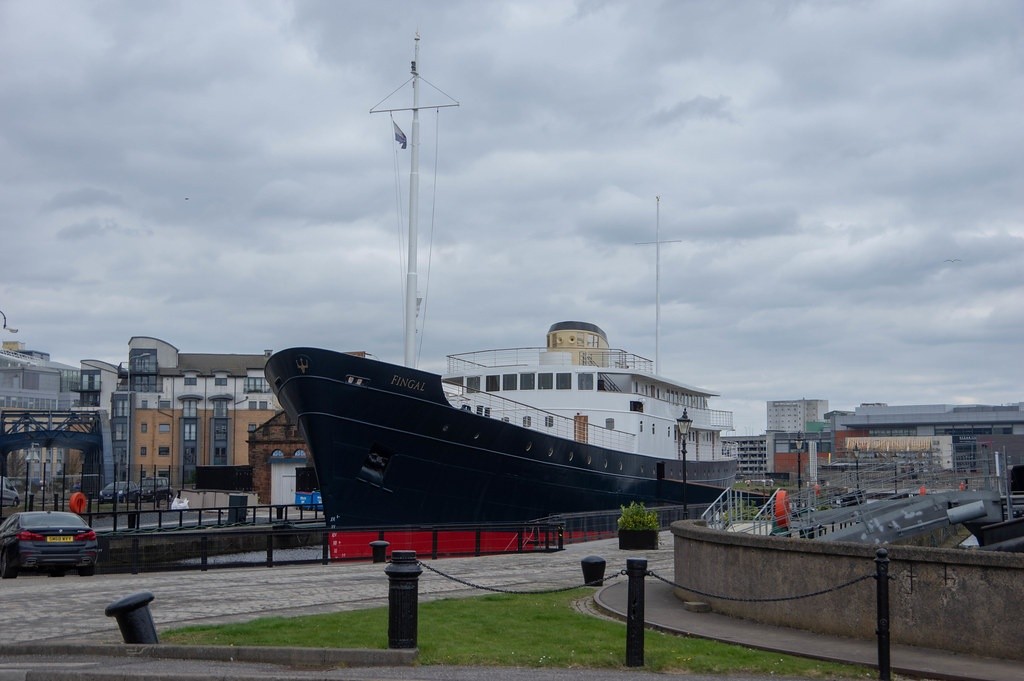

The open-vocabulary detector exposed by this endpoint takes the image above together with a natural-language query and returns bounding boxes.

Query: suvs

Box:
[138,477,173,500]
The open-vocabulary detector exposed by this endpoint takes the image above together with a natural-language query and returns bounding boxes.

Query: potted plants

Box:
[617,500,662,551]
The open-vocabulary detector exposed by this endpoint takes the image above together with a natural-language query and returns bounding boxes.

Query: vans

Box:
[0,476,20,507]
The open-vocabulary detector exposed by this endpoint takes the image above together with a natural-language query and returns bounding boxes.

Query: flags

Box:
[393,121,407,149]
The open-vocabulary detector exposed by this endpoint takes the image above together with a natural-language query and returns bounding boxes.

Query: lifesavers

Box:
[775,490,790,527]
[815,484,821,495]
[960,482,965,491]
[69,491,87,513]
[920,486,926,496]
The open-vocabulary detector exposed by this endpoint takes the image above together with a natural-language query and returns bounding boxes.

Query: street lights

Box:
[793,432,804,507]
[853,442,860,493]
[892,452,898,494]
[126,352,151,481]
[676,407,693,519]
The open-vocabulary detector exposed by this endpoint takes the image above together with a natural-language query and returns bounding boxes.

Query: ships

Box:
[264,30,773,558]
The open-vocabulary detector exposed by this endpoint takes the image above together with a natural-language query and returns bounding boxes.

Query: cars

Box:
[100,481,140,504]
[0,510,99,579]
